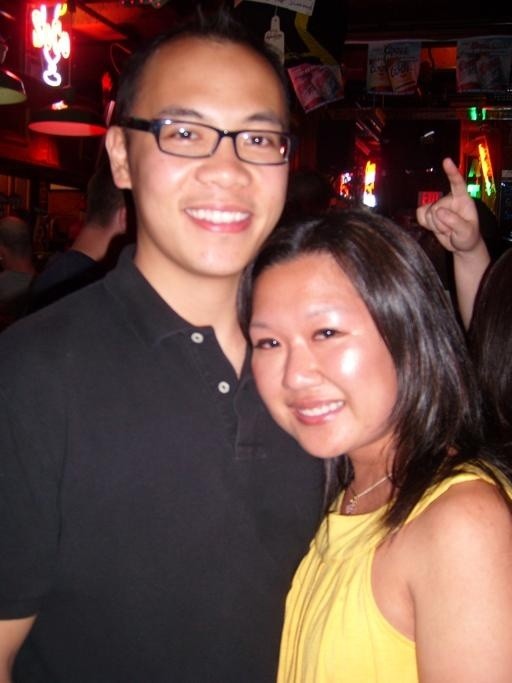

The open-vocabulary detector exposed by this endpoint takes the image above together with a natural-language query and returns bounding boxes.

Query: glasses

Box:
[119,117,293,166]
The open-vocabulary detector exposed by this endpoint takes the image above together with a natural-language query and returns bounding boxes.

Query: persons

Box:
[1,10,511,682]
